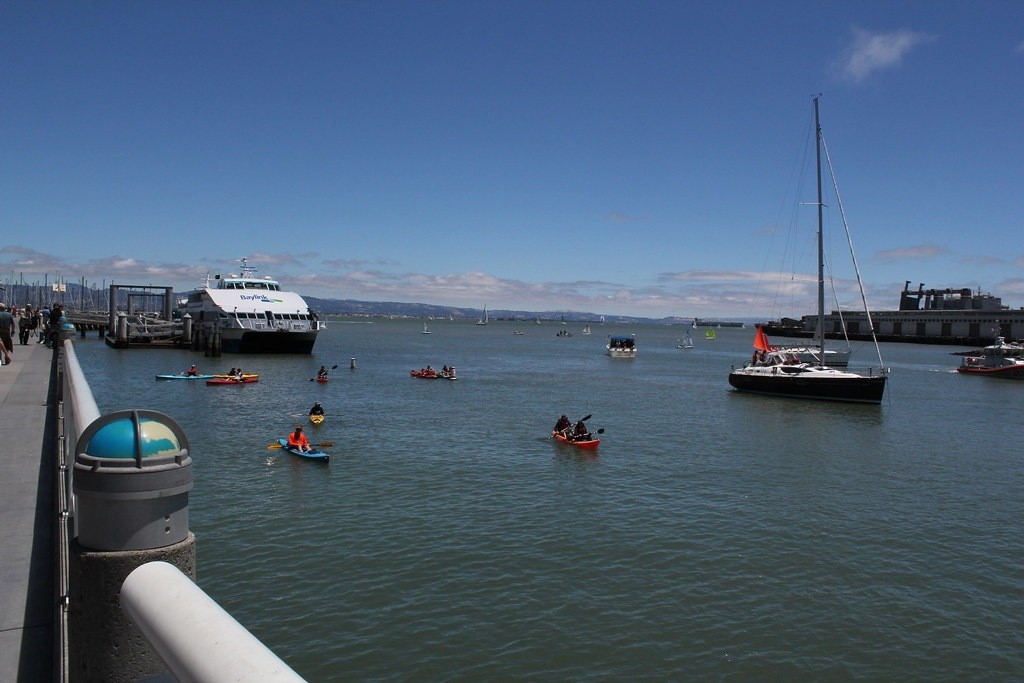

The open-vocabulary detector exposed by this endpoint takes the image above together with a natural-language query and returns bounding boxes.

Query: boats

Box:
[277,438,330,460]
[173,256,320,356]
[206,374,259,386]
[410,373,438,379]
[316,376,328,383]
[310,415,325,424]
[956,355,1024,378]
[156,373,216,378]
[552,431,601,449]
[984,337,1024,355]
[606,333,638,358]
[436,374,455,378]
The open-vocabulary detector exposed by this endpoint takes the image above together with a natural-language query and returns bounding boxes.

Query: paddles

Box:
[547,415,591,440]
[295,414,344,416]
[309,365,337,381]
[566,429,604,441]
[267,442,333,450]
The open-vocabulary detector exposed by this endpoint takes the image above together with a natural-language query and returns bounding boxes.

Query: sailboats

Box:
[421,322,431,334]
[676,318,746,350]
[320,314,328,329]
[476,304,489,325]
[727,93,889,402]
[511,313,592,337]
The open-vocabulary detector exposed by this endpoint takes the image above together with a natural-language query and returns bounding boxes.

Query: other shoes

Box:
[19,338,23,344]
[23,343,28,345]
[37,341,41,343]
[5,357,11,364]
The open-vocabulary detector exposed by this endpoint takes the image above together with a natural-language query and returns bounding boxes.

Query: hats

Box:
[296,424,302,430]
[192,364,195,368]
[315,401,322,405]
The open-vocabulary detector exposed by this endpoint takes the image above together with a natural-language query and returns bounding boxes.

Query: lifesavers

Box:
[968,357,972,364]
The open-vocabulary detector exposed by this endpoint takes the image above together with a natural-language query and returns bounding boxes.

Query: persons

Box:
[442,365,448,373]
[0,303,15,364]
[318,365,327,375]
[309,401,325,416]
[18,304,34,346]
[12,305,16,318]
[554,415,587,441]
[760,350,767,362]
[752,350,759,366]
[421,365,435,375]
[230,367,243,381]
[37,303,65,349]
[188,365,196,376]
[287,425,311,452]
